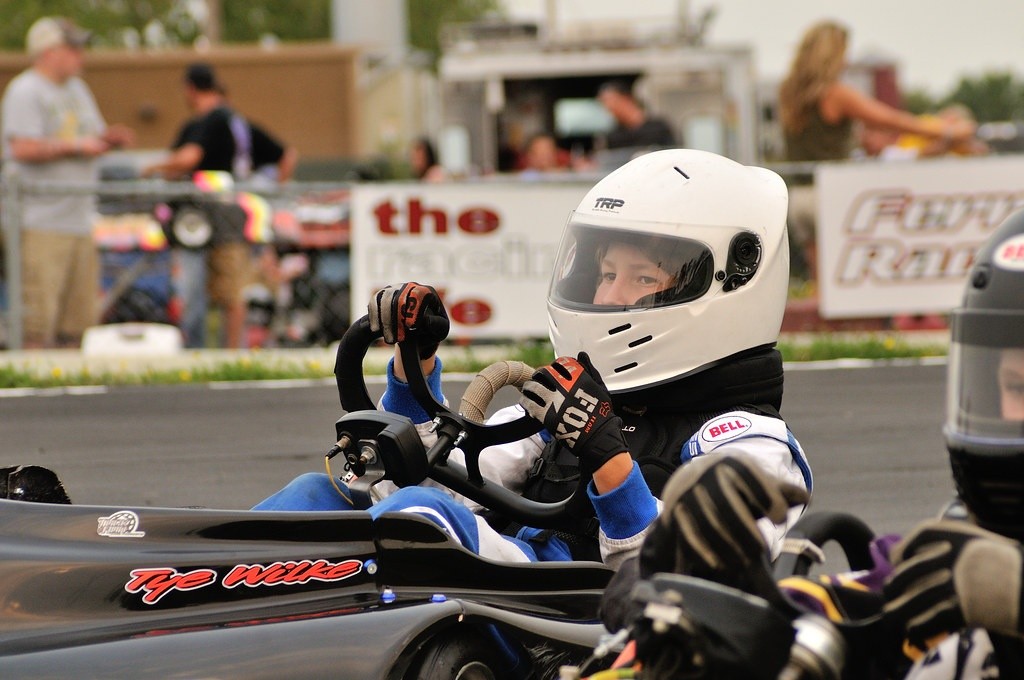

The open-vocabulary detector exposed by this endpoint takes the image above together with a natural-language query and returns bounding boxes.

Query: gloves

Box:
[653,444,812,571]
[874,508,1024,650]
[516,346,630,473]
[370,280,450,364]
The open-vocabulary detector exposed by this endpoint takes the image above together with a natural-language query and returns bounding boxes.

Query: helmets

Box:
[947,201,1023,538]
[541,143,792,399]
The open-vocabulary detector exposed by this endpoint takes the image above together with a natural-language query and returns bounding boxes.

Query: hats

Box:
[23,7,90,56]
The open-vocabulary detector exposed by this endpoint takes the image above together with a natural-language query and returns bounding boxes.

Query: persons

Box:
[166,88,230,344]
[775,18,976,280]
[642,194,1024,680]
[513,142,576,180]
[241,147,813,679]
[599,86,679,164]
[851,122,963,161]
[402,139,449,180]
[169,62,292,346]
[0,17,133,347]
